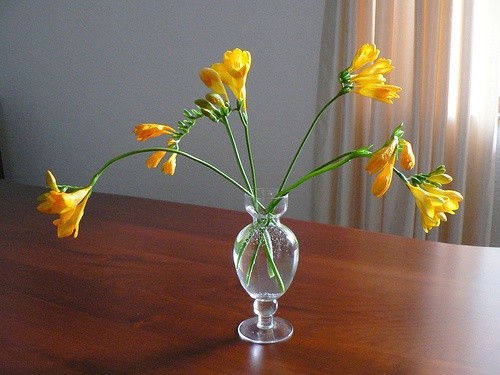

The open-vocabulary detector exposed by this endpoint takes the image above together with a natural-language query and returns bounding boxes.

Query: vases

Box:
[231,188,299,345]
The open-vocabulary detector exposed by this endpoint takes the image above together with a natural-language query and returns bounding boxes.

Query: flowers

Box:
[36,43,463,294]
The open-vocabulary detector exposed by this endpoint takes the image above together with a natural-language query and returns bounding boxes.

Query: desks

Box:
[1,179,499,374]
